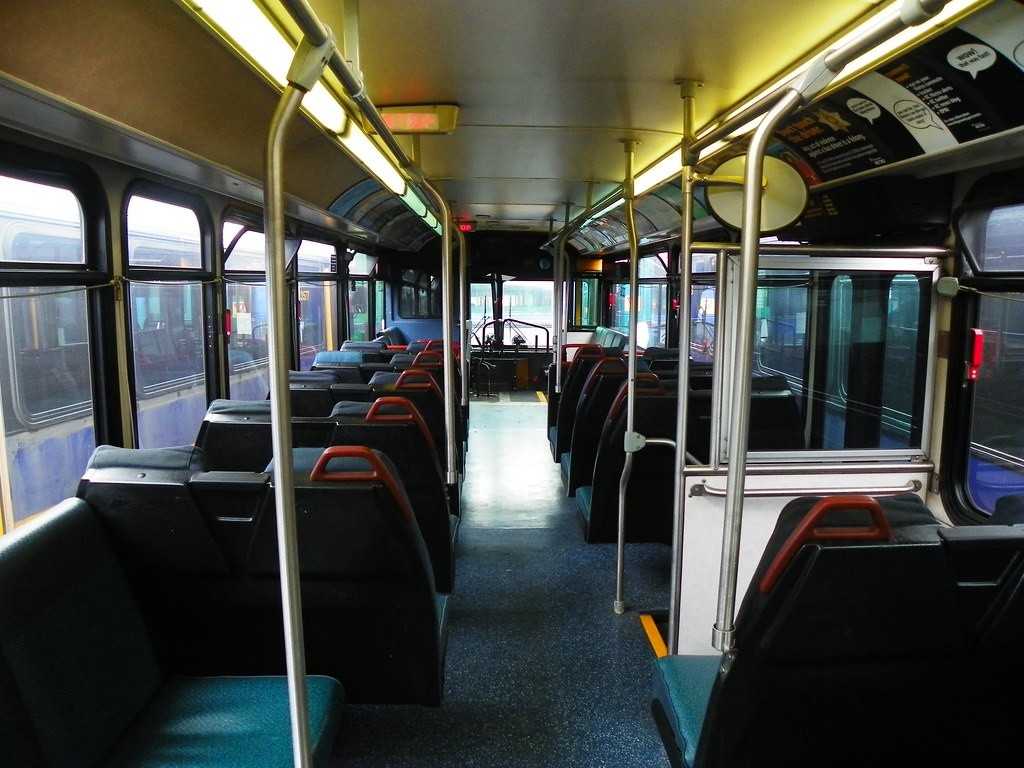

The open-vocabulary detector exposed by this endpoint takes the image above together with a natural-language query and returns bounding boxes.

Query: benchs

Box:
[0,500,347,768]
[76,325,804,710]
[648,492,1024,768]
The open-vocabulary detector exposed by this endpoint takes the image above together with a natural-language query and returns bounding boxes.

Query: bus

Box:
[654,201,1023,513]
[1,175,379,533]
[471,284,551,328]
